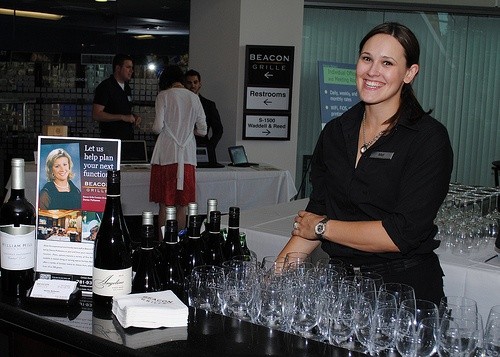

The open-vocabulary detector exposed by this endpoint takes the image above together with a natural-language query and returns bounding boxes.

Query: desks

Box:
[5,161,299,215]
[200,196,500,347]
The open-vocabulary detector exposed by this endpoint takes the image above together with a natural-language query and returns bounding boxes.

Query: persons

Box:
[184,70,224,168]
[92,54,142,163]
[40,149,81,211]
[149,66,208,240]
[266,22,453,331]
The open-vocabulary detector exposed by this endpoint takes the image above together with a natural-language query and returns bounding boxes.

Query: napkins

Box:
[111,289,189,329]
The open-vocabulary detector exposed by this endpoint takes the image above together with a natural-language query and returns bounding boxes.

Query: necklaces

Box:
[360,113,390,154]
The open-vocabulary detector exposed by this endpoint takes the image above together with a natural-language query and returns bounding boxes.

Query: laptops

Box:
[228,146,259,167]
[121,140,151,167]
[196,143,224,168]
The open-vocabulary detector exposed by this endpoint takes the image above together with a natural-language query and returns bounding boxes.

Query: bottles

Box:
[91,170,134,305]
[92,300,123,344]
[0,159,36,306]
[132,198,251,307]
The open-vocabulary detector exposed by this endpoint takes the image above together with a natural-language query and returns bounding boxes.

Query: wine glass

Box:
[435,183,500,256]
[188,251,500,357]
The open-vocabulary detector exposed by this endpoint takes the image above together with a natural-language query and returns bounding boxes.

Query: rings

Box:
[293,222,298,229]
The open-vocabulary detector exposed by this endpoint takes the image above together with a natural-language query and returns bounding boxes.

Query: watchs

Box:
[315,217,330,240]
